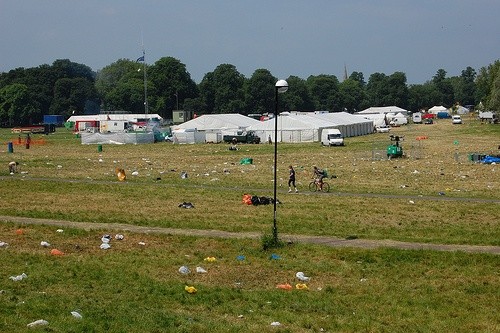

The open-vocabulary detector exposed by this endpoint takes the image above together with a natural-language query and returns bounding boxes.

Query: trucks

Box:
[437,112,451,119]
[413,113,422,123]
[479,112,498,121]
[223,130,262,144]
[389,118,408,127]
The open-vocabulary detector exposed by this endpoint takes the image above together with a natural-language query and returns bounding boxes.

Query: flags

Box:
[137,56,144,61]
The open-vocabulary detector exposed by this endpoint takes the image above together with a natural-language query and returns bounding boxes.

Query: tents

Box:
[428,106,450,114]
[245,112,373,143]
[171,114,262,143]
[355,106,407,119]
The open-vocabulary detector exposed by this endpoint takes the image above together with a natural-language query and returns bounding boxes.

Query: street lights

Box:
[273,79,288,240]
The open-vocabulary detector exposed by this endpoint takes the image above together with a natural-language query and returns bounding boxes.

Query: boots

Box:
[288,187,291,193]
[295,188,298,193]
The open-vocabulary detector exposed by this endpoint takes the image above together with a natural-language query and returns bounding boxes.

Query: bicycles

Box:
[309,177,330,193]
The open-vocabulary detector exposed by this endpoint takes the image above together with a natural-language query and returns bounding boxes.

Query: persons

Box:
[288,165,298,193]
[25,134,31,144]
[311,166,325,185]
[8,161,19,174]
[374,125,377,132]
[268,135,272,144]
[18,135,21,144]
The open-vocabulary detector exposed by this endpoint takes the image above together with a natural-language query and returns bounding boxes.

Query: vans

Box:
[320,128,344,147]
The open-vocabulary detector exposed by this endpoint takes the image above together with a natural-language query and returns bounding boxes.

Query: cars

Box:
[451,115,461,124]
[425,120,433,124]
[375,125,391,133]
[423,114,433,120]
